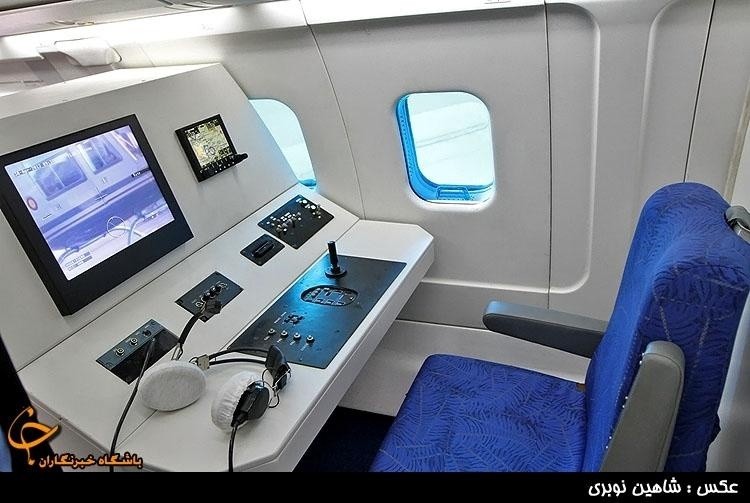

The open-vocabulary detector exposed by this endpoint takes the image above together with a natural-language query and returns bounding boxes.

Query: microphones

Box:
[171,298,221,360]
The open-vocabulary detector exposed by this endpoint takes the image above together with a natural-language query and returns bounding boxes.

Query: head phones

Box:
[136,344,292,434]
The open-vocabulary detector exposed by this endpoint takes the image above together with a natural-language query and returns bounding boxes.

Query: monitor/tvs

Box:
[0,113,195,316]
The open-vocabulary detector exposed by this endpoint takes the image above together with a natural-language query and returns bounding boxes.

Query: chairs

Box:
[366,181,750,472]
